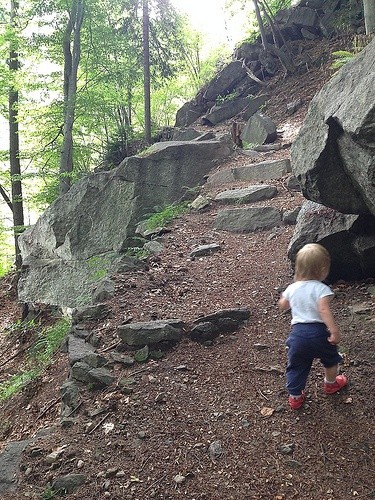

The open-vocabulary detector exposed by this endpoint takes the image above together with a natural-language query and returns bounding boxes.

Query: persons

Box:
[278,243,347,409]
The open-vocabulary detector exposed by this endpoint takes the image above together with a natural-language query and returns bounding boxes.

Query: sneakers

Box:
[289,391,304,409]
[324,375,346,393]
